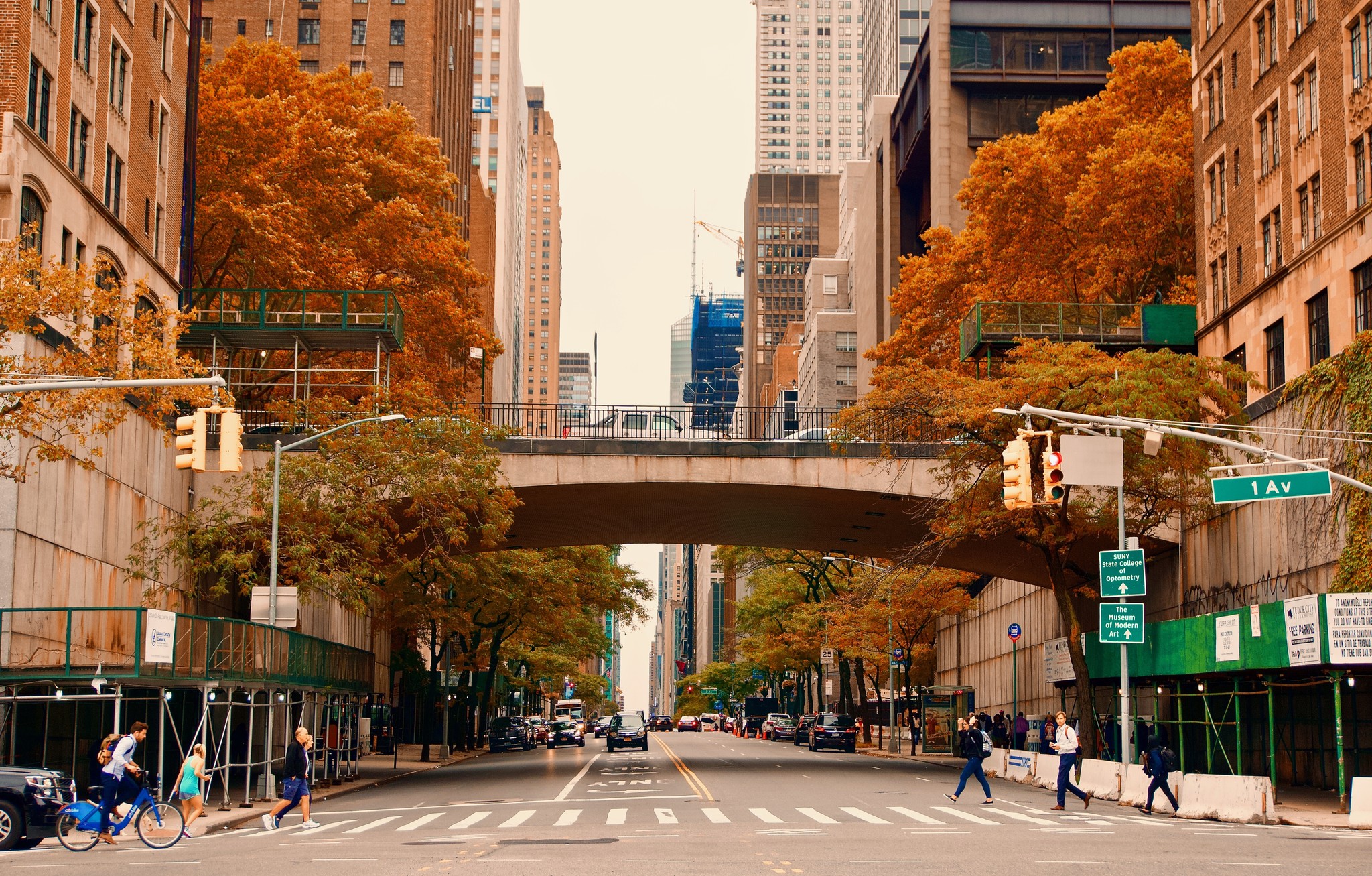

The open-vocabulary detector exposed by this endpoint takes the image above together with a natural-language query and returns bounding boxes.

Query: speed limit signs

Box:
[820,643,834,659]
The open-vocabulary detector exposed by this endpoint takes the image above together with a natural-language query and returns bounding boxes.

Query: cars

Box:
[587,710,673,752]
[561,411,727,439]
[242,421,325,450]
[0,765,76,851]
[724,722,733,733]
[488,699,587,753]
[942,430,990,446]
[771,426,864,442]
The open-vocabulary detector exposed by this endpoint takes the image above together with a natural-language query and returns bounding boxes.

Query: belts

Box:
[1060,753,1075,756]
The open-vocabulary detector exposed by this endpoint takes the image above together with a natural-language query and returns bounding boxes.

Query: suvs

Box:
[744,714,857,754]
[415,415,497,442]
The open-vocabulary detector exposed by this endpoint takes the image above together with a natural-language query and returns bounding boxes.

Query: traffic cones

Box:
[762,726,768,740]
[743,727,749,738]
[755,729,761,739]
[731,725,736,735]
[736,727,741,737]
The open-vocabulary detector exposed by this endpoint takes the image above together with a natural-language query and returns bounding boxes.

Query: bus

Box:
[677,713,720,732]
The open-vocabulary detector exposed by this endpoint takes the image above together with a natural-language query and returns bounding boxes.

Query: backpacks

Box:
[967,728,993,758]
[97,733,134,765]
[1057,725,1082,755]
[1149,746,1179,772]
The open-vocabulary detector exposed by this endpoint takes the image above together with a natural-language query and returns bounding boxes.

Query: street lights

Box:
[788,567,829,712]
[823,557,899,746]
[255,414,405,801]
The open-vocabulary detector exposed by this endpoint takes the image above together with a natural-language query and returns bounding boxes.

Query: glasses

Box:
[963,722,969,726]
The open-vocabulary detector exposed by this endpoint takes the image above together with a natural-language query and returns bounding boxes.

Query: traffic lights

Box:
[570,683,574,690]
[688,687,692,690]
[218,411,243,471]
[174,410,205,470]
[1040,449,1066,503]
[575,683,577,691]
[987,408,1131,774]
[1001,439,1033,510]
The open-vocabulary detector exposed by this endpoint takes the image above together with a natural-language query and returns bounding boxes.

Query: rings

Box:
[206,780,207,781]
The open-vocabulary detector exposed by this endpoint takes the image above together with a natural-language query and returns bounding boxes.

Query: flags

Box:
[606,666,610,678]
[676,660,685,672]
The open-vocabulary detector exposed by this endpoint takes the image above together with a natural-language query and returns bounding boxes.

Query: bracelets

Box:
[969,723,973,724]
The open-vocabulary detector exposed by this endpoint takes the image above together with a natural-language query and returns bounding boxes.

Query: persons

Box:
[797,713,801,721]
[927,714,936,743]
[1132,717,1172,764]
[943,712,993,805]
[1039,714,1058,755]
[262,726,320,830]
[855,719,860,735]
[173,743,210,838]
[913,713,920,745]
[813,710,818,715]
[98,721,148,845]
[945,715,950,745]
[1075,713,1122,763]
[984,710,1029,751]
[1138,734,1179,818]
[1050,711,1091,811]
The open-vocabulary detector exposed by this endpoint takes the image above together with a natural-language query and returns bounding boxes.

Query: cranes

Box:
[693,219,745,278]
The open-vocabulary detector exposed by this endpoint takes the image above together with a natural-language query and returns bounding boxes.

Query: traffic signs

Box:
[784,699,794,702]
[1099,547,1147,646]
[728,699,737,702]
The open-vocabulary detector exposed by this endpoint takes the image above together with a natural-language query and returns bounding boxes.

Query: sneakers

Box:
[179,825,193,838]
[262,814,273,830]
[302,819,320,828]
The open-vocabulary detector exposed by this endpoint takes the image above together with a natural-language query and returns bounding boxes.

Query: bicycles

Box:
[54,769,185,851]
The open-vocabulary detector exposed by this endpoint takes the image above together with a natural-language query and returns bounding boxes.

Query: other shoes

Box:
[273,814,281,828]
[1083,794,1091,809]
[1050,803,1064,811]
[1138,808,1151,815]
[978,801,993,805]
[98,832,118,845]
[311,819,319,823]
[110,807,124,818]
[1171,813,1177,818]
[943,793,956,803]
